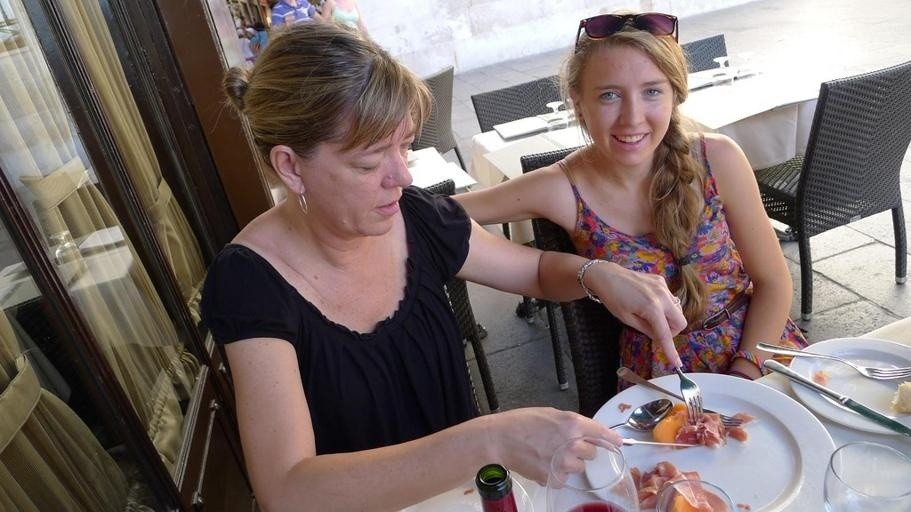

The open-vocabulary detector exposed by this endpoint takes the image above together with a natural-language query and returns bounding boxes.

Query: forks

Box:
[674,368,703,425]
[756,341,911,382]
[616,367,743,431]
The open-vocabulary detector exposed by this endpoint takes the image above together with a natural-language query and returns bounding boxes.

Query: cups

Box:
[654,478,734,512]
[821,440,911,512]
[545,435,641,512]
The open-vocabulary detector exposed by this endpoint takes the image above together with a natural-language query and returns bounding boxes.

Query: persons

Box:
[249,21,271,57]
[311,0,371,39]
[199,16,690,512]
[234,26,257,66]
[269,0,320,34]
[435,12,810,395]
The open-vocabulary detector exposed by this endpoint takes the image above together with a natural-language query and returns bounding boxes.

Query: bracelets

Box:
[575,257,612,305]
[723,370,754,382]
[727,352,768,376]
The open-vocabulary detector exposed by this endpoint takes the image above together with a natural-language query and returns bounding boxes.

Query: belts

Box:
[679,289,751,335]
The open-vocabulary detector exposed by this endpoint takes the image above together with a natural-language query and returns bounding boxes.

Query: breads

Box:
[890,381,911,413]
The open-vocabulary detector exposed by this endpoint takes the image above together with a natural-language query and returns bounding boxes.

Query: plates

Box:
[686,59,756,92]
[0,259,80,311]
[788,337,911,435]
[79,225,124,249]
[580,372,837,512]
[493,115,551,139]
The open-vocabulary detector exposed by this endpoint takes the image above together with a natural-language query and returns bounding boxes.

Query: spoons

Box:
[609,398,673,432]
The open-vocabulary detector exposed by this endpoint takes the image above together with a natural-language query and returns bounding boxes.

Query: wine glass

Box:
[542,101,571,137]
[49,230,89,282]
[712,49,735,88]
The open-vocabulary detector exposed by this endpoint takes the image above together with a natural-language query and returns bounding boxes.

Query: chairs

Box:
[470,75,561,133]
[412,66,468,172]
[520,144,586,390]
[755,60,909,322]
[678,35,729,72]
[32,183,116,247]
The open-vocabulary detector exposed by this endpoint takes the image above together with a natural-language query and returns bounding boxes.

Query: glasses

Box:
[575,13,680,52]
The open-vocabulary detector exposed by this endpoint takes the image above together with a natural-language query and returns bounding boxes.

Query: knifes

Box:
[623,437,695,447]
[762,358,911,439]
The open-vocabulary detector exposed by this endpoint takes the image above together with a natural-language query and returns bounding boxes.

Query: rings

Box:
[675,296,682,306]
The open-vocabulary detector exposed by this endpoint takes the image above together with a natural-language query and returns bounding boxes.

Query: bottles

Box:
[474,462,518,512]
